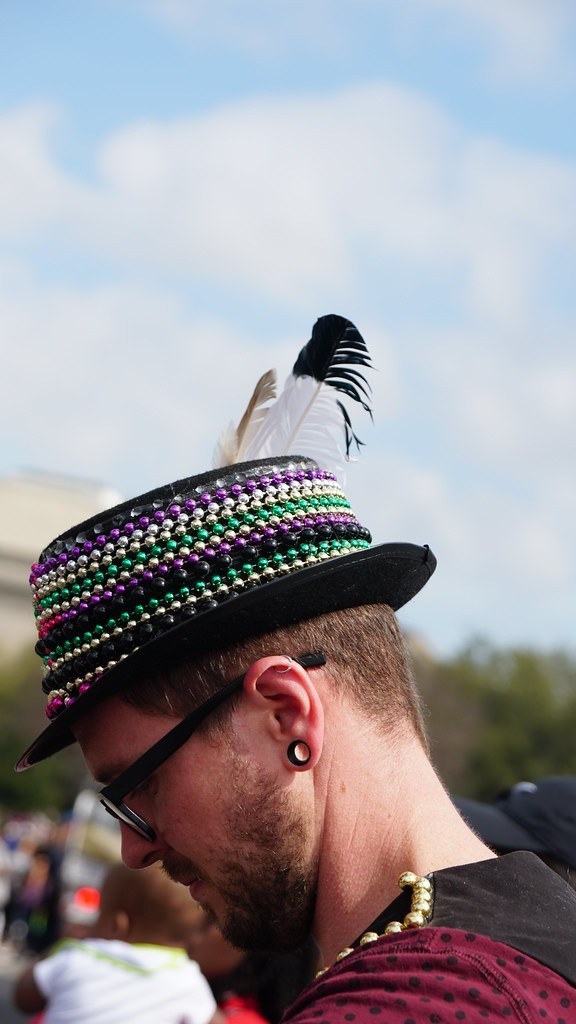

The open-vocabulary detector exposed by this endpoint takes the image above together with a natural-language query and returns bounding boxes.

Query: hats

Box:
[453,776,576,859]
[12,313,438,772]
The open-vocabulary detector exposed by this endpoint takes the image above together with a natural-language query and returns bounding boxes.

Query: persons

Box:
[0,775,576,1024]
[16,457,576,1024]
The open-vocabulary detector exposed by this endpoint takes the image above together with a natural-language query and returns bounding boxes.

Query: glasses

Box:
[93,648,328,844]
